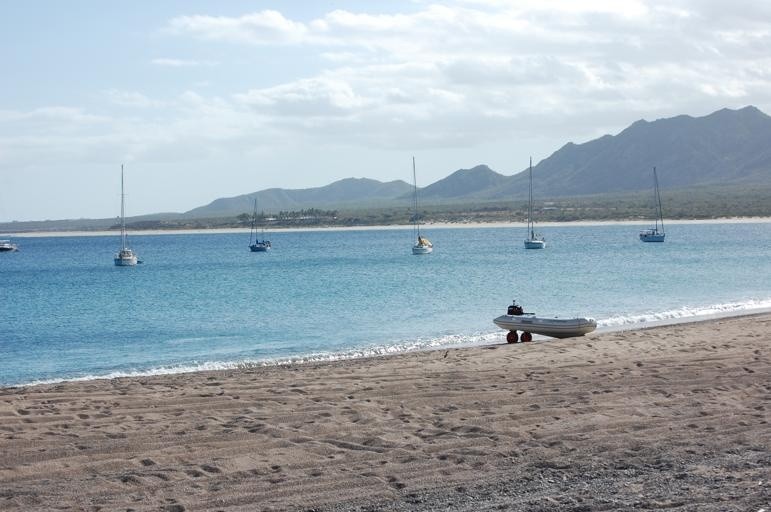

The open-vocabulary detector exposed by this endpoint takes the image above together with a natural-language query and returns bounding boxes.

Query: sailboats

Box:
[639,165,666,243]
[114,162,140,267]
[247,197,273,254]
[410,155,434,255]
[523,154,547,251]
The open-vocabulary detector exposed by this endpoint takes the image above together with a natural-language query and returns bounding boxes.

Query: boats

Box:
[491,312,600,339]
[0,239,19,253]
